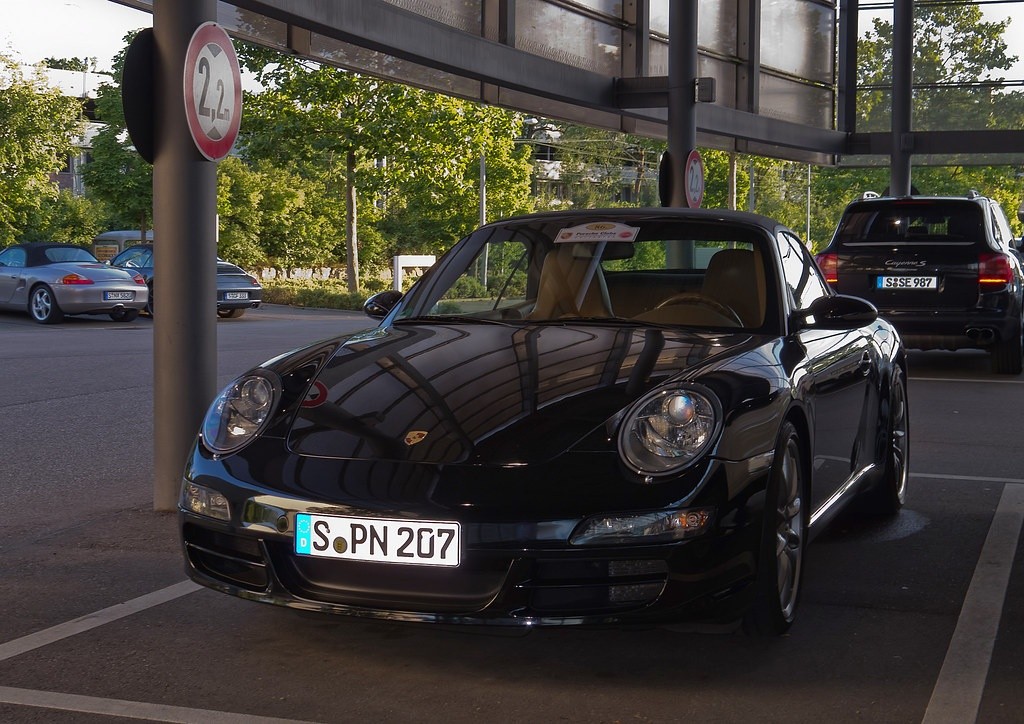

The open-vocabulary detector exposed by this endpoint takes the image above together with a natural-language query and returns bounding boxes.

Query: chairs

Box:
[523,252,607,320]
[701,248,765,328]
[868,220,899,242]
[946,216,975,241]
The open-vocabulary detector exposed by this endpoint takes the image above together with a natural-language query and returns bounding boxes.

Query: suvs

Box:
[815,190,1024,372]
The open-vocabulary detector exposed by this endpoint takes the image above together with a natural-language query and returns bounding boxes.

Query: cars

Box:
[146,263,262,318]
[0,241,149,324]
[101,243,157,291]
[176,206,911,639]
